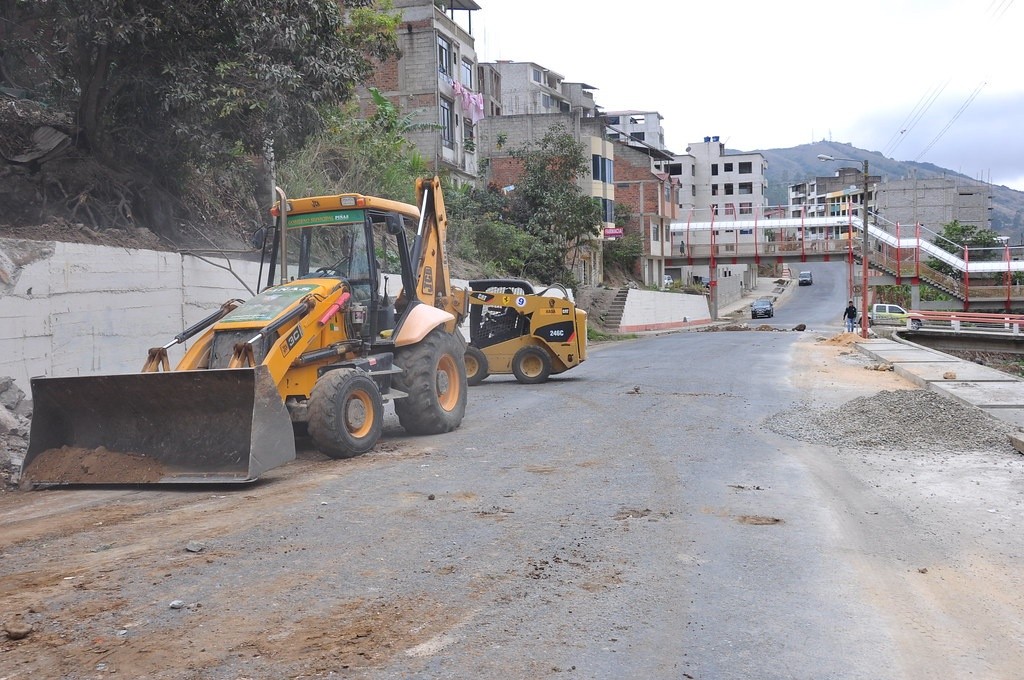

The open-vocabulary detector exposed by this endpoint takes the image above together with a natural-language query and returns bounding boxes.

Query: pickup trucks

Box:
[857,304,926,331]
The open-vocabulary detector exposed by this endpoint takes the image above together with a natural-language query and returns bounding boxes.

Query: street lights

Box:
[819,154,870,337]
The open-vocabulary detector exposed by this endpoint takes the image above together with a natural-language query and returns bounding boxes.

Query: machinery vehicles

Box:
[450,279,589,389]
[14,176,469,486]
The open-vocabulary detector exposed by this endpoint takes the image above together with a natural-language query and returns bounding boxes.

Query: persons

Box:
[480,289,519,338]
[333,235,378,305]
[843,301,857,332]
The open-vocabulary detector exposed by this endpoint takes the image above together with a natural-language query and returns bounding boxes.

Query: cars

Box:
[798,271,813,286]
[695,276,710,288]
[664,275,673,288]
[749,299,774,318]
